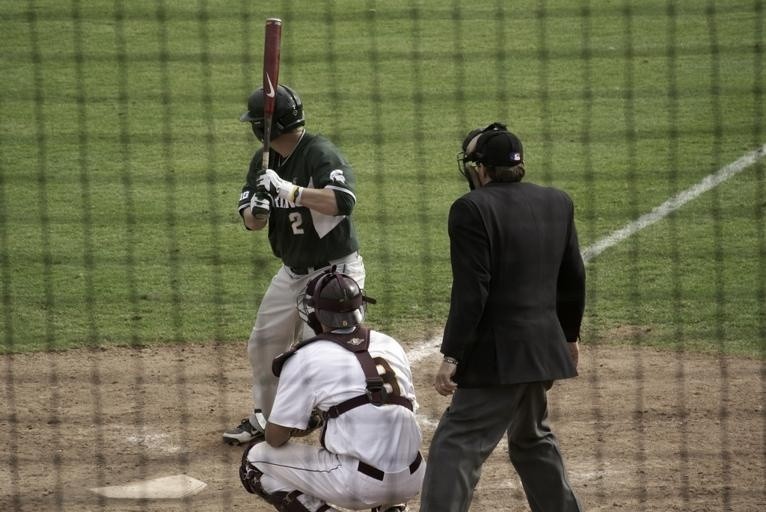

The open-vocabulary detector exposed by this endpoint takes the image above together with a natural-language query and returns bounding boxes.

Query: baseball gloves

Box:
[291,405,325,436]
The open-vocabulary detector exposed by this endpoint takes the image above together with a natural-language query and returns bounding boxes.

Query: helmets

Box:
[462,123,523,166]
[240,84,304,129]
[305,264,364,328]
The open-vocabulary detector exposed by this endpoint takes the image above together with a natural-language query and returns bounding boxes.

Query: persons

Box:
[239,265,427,512]
[420,122,587,512]
[222,84,366,446]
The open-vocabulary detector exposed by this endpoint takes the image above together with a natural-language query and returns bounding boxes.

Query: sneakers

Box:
[223,418,264,446]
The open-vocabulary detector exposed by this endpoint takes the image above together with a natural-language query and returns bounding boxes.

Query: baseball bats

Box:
[255,18,282,217]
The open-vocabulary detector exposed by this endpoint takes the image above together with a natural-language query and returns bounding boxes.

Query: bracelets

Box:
[443,358,458,365]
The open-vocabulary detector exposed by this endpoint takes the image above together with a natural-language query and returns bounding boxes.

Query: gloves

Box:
[251,169,303,218]
